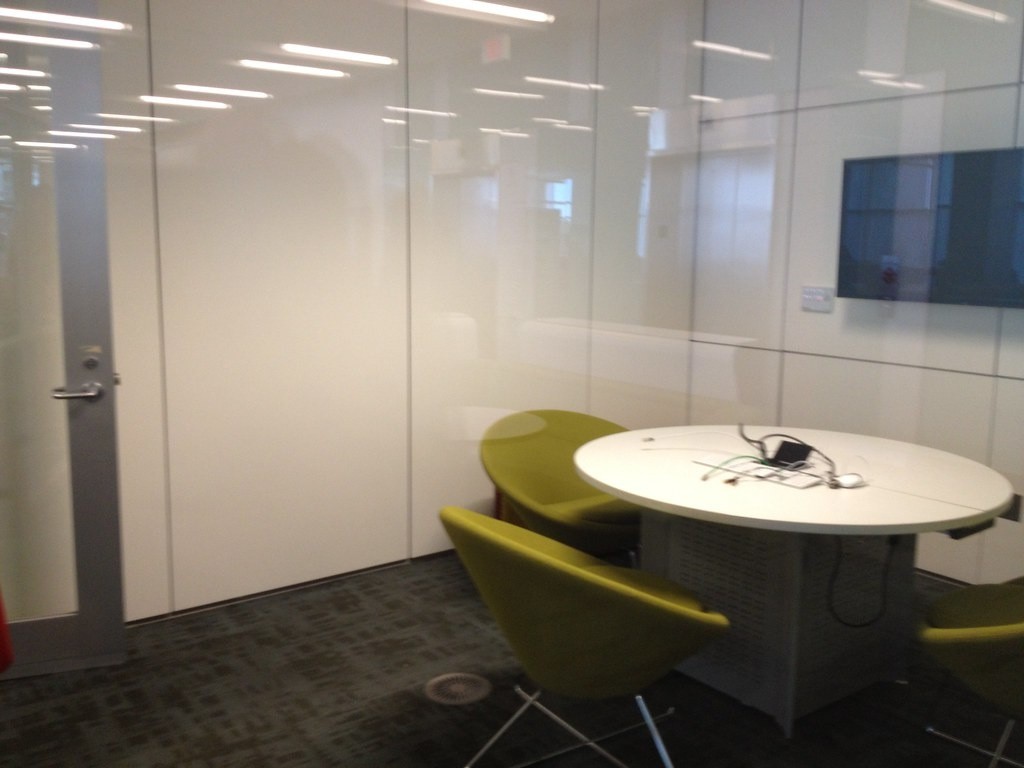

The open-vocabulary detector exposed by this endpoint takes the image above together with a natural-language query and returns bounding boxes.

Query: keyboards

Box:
[694,451,821,488]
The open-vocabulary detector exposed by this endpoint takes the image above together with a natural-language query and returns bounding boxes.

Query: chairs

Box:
[910,574,1024,768]
[481,408,644,564]
[440,507,731,768]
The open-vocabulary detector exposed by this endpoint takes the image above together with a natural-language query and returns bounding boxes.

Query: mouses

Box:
[837,473,866,489]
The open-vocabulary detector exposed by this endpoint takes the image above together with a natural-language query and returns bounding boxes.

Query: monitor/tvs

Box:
[839,146,1024,309]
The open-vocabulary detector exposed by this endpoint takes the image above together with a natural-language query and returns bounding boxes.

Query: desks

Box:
[575,426,1015,737]
